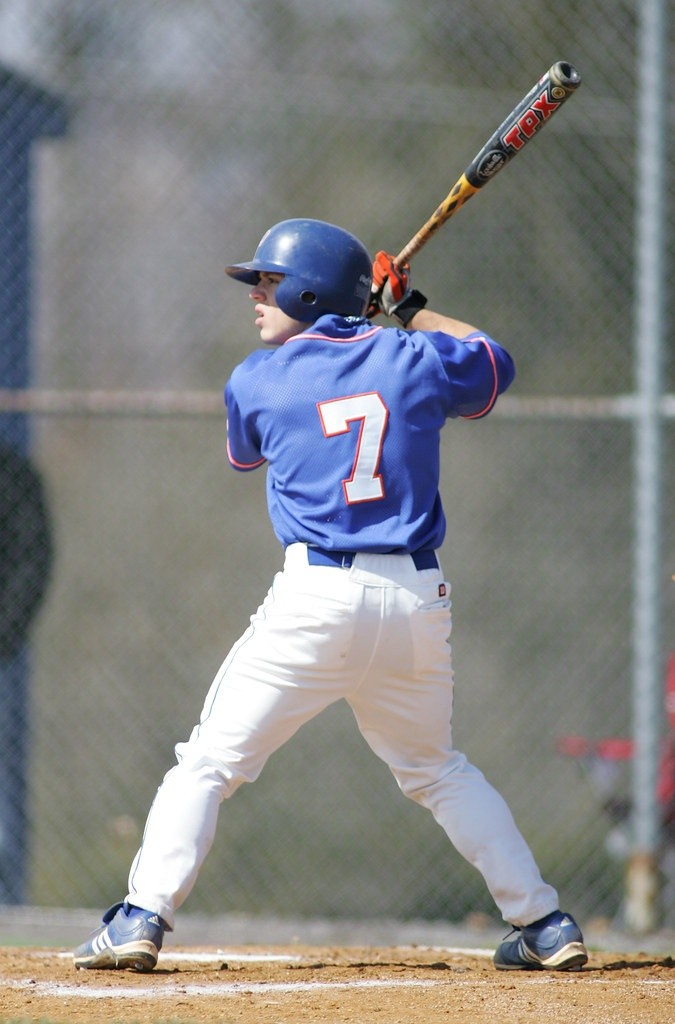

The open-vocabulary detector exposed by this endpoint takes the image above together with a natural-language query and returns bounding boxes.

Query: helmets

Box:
[225,218,373,322]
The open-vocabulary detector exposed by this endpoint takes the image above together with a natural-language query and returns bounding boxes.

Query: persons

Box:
[74,219,587,969]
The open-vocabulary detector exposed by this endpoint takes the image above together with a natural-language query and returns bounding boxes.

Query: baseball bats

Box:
[392,59,584,271]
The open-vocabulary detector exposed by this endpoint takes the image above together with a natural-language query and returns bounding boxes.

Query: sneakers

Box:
[73,903,164,970]
[494,913,588,970]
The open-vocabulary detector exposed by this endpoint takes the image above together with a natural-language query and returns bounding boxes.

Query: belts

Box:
[307,546,438,571]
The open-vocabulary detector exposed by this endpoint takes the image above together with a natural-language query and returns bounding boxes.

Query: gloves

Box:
[366,250,427,328]
